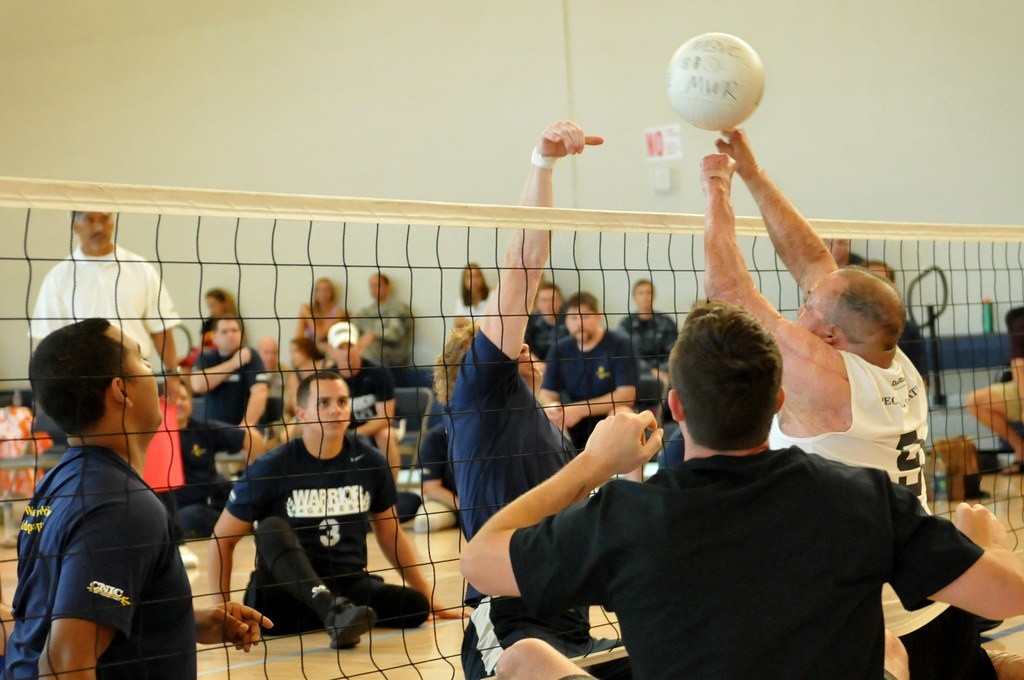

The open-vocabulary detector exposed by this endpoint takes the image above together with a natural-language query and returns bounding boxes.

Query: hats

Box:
[328,321,359,349]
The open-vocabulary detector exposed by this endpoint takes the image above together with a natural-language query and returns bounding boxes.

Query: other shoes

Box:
[177,545,198,569]
[1000,460,1024,475]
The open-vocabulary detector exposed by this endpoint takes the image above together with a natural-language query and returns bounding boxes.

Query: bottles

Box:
[981,297,993,335]
[930,452,948,509]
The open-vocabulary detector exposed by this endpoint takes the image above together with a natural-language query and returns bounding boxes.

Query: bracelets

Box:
[532,150,559,172]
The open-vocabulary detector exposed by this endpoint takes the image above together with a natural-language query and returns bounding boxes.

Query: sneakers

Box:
[325,598,376,648]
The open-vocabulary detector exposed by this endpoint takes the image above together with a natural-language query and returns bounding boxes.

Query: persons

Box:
[823,237,930,394]
[207,372,471,649]
[159,273,423,539]
[414,264,687,535]
[433,121,624,680]
[27,212,198,570]
[458,301,1024,679]
[0,317,274,680]
[965,304,1024,477]
[699,128,1004,680]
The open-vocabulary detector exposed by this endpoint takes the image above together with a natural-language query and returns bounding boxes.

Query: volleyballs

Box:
[663,31,768,132]
